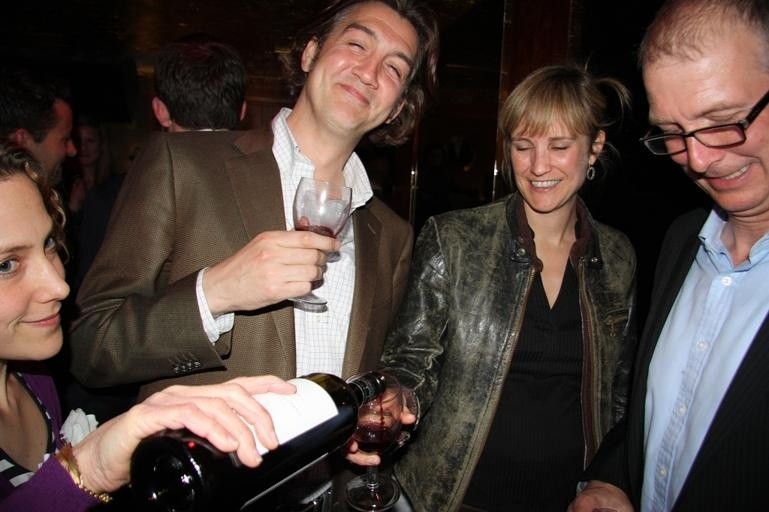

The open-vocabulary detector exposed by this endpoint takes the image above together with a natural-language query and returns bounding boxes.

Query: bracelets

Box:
[57,444,111,502]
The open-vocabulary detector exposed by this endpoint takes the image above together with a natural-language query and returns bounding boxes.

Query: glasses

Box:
[638,89,769,158]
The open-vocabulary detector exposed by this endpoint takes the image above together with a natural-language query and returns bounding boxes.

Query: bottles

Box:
[130,371,394,512]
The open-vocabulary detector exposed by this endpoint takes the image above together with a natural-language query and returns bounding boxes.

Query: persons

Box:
[348,67,636,511]
[0,139,297,512]
[574,0,769,512]
[2,34,246,285]
[72,2,434,511]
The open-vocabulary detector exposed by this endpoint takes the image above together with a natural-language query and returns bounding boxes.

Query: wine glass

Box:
[355,405,396,511]
[288,178,356,312]
